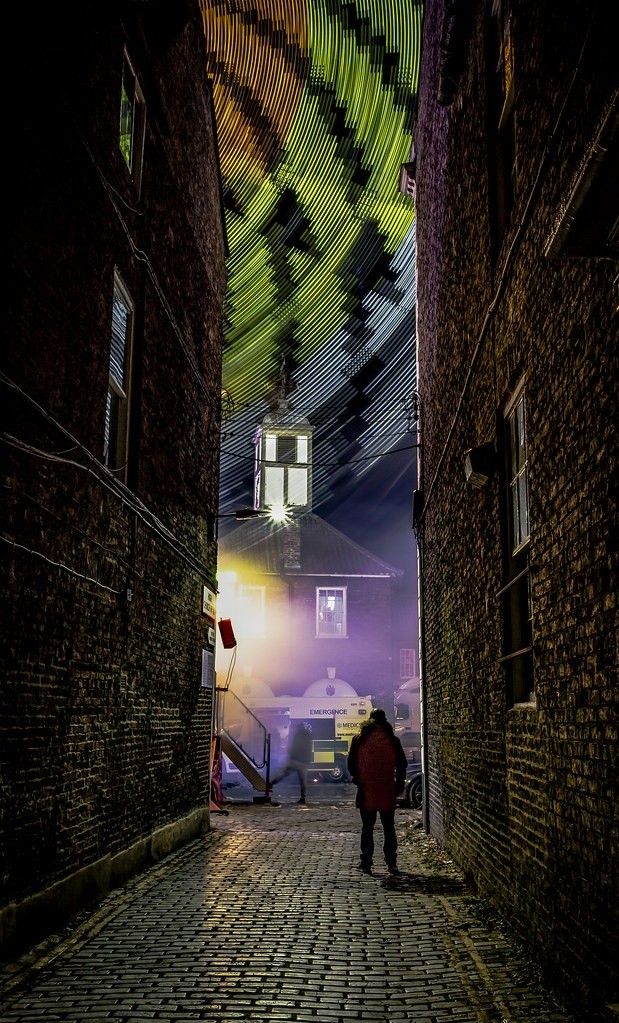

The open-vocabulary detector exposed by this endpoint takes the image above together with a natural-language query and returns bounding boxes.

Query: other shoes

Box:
[356,864,371,872]
[387,866,398,875]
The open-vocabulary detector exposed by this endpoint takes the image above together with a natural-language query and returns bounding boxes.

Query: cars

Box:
[389,726,423,810]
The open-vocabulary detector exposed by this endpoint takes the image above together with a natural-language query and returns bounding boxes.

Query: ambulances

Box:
[220,695,375,783]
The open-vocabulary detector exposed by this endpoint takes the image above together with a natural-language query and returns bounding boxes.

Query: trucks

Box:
[393,677,423,731]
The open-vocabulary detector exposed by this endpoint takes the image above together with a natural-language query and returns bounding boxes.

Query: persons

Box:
[270,724,313,805]
[346,707,408,872]
